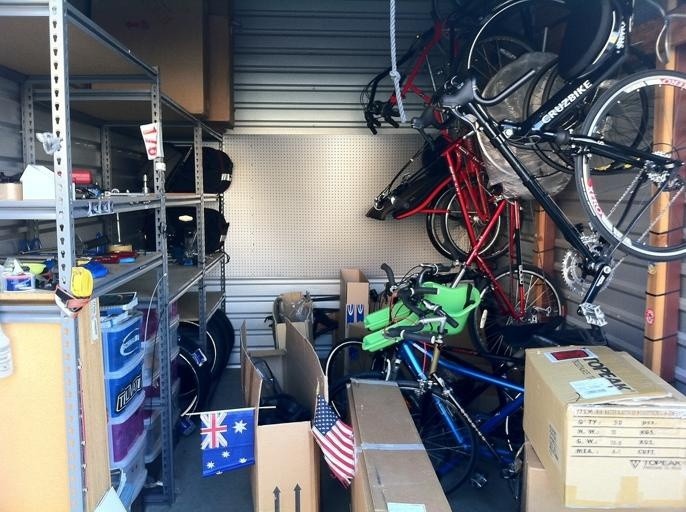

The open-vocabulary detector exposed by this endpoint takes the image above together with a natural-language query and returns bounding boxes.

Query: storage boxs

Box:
[269,287,314,350]
[97,284,184,510]
[522,342,686,512]
[82,2,213,117]
[342,370,454,512]
[233,311,331,511]
[520,435,561,511]
[337,263,378,374]
[201,0,238,128]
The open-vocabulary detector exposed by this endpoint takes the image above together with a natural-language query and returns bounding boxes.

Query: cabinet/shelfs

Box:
[1,2,175,512]
[31,83,233,417]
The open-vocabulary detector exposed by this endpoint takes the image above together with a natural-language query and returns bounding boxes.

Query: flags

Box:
[310,388,357,490]
[196,410,257,478]
